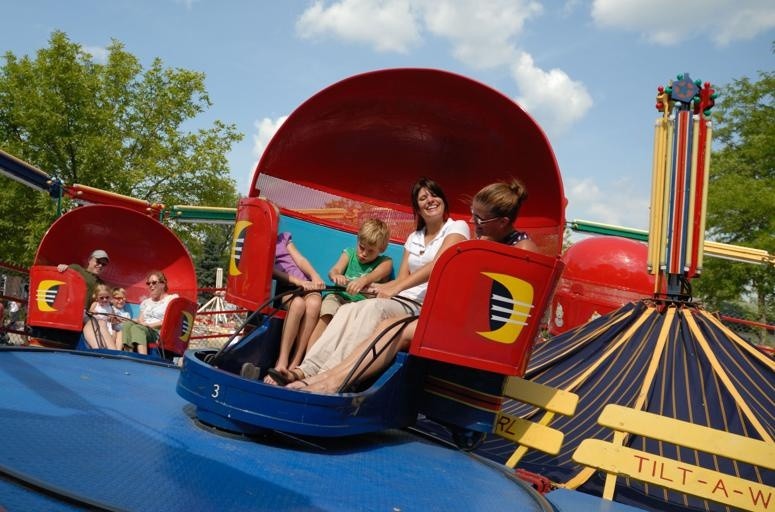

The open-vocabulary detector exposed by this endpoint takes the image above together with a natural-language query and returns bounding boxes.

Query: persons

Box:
[239,216,393,388]
[0,292,27,345]
[265,175,472,390]
[282,176,541,392]
[56,248,180,355]
[253,195,326,389]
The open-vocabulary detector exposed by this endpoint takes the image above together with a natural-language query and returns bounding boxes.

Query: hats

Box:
[90,249,111,261]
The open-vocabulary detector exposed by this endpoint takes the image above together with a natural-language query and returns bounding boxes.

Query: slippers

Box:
[267,368,301,384]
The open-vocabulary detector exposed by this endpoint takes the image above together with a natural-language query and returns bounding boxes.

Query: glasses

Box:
[96,261,108,266]
[146,281,158,286]
[114,296,124,299]
[470,208,500,225]
[100,296,110,299]
